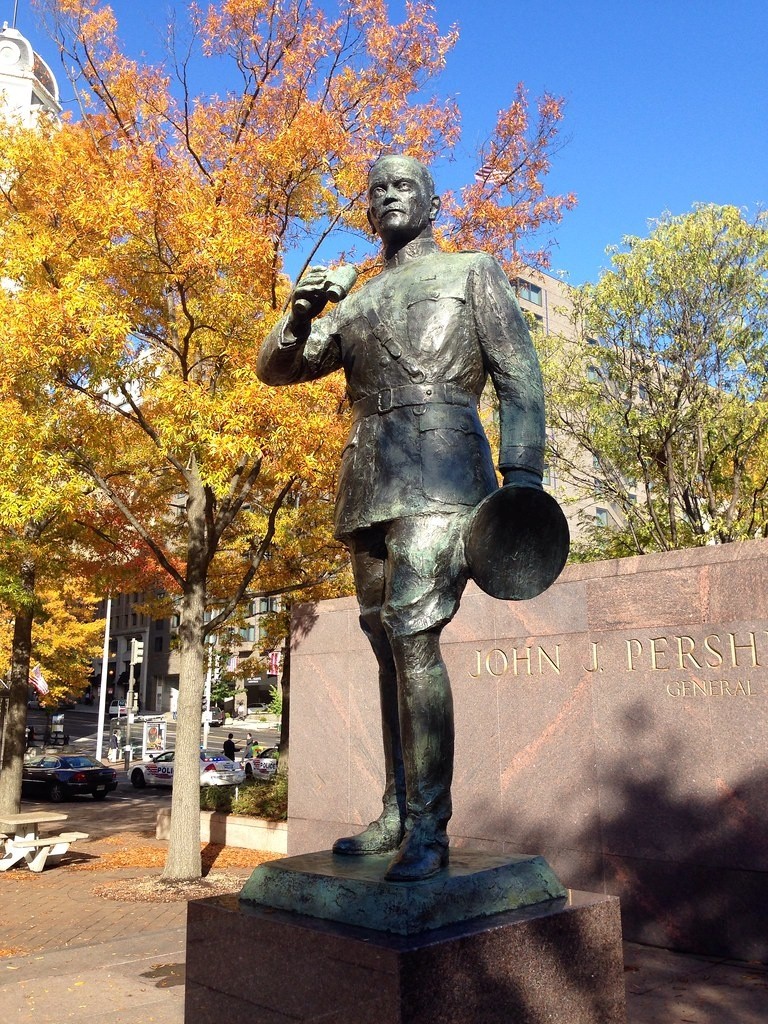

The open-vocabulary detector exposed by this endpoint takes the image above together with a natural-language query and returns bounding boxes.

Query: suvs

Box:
[109,699,127,720]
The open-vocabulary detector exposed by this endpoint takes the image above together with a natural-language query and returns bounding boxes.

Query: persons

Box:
[222,733,243,760]
[25,726,34,746]
[243,734,258,759]
[107,730,119,763]
[258,151,548,877]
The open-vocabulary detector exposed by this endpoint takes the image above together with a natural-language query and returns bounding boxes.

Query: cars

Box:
[21,754,118,803]
[245,745,280,783]
[127,747,247,792]
[247,703,268,715]
[209,706,224,727]
